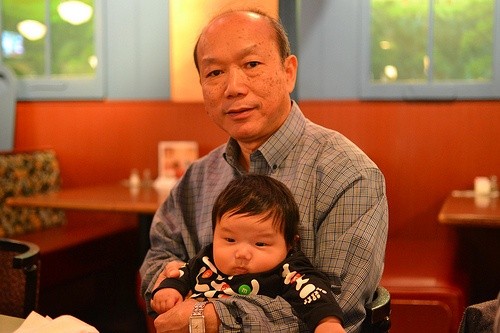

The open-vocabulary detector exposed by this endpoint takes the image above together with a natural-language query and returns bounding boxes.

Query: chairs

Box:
[0,238,42,318]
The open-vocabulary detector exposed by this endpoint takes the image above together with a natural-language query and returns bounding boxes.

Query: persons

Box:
[150,173,347,333]
[140,8,389,333]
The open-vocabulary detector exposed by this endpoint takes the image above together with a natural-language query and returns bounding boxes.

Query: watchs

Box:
[188,301,209,333]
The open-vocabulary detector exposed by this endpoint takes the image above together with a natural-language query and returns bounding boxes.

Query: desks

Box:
[437,190,500,301]
[6,178,168,228]
